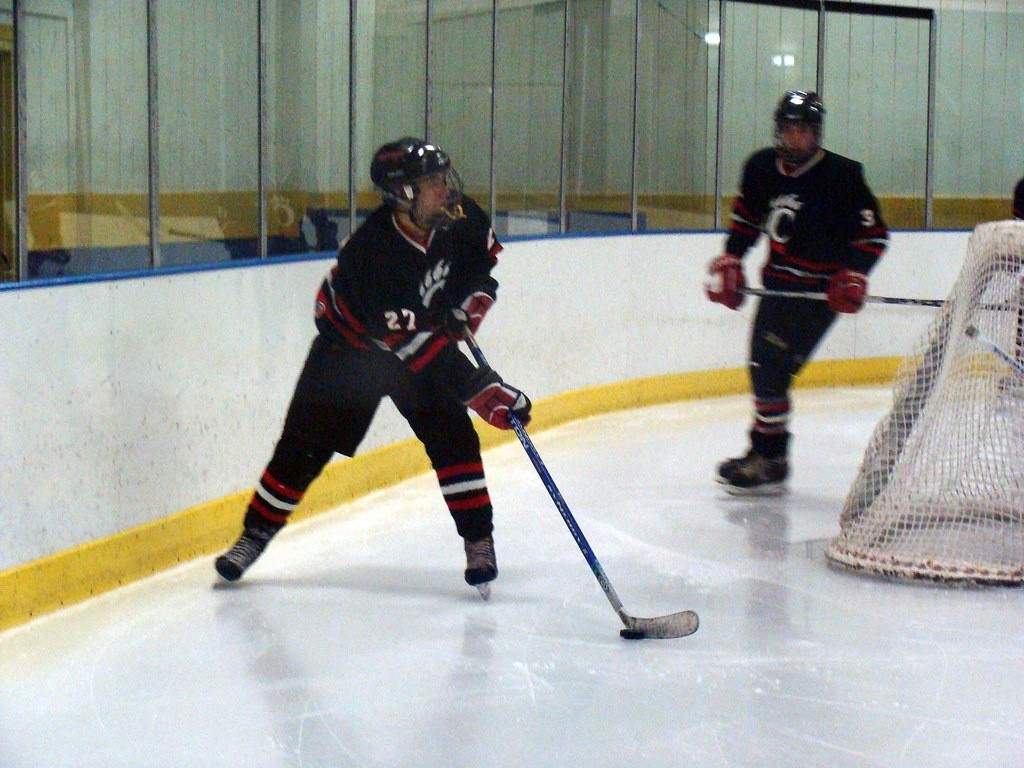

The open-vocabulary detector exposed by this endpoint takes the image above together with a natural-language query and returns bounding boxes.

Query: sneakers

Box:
[715,431,789,496]
[215,504,285,589]
[450,505,497,601]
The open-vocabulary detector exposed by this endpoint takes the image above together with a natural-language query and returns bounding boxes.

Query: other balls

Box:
[618,628,643,639]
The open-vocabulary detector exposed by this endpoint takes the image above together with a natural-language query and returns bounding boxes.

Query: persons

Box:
[702,90,890,496]
[214,155,339,258]
[215,136,532,601]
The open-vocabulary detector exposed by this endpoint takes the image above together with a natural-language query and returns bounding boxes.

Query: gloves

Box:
[448,364,532,430]
[826,267,867,313]
[708,254,745,310]
[443,268,499,341]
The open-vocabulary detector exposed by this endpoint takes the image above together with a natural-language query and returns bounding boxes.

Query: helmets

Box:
[370,137,464,231]
[773,89,824,162]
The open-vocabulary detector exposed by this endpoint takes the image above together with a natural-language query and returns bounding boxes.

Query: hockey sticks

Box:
[967,325,1023,378]
[464,324,699,640]
[740,281,1013,312]
